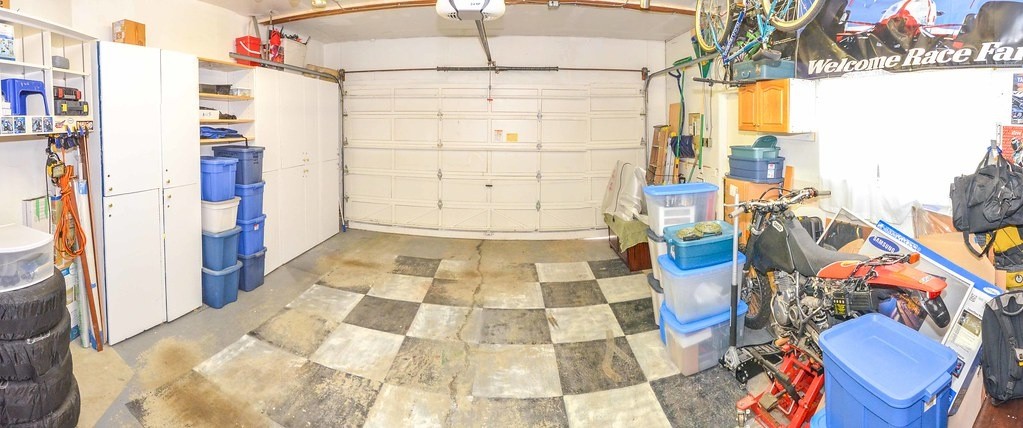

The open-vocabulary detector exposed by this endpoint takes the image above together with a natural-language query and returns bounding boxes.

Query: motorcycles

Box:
[721,186,952,366]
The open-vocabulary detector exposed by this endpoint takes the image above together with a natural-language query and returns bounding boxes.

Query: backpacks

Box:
[981,291,1023,406]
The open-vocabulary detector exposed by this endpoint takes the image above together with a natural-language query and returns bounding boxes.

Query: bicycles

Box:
[694,0,828,54]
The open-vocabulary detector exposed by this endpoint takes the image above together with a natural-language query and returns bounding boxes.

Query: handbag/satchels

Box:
[950,147,1023,258]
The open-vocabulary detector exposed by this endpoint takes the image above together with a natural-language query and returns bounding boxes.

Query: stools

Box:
[1,78,50,116]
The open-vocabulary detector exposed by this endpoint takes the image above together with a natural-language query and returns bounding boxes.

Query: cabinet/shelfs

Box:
[198,56,255,143]
[88,41,204,346]
[228,66,340,275]
[737,77,815,134]
[0,6,98,136]
[604,213,651,272]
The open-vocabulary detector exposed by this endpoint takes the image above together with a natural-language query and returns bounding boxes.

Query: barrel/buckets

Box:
[236,36,262,66]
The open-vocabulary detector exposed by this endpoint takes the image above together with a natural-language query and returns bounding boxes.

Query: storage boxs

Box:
[211,145,265,184]
[658,251,747,323]
[641,182,719,237]
[199,83,233,95]
[201,260,243,309]
[66,301,80,341]
[200,155,239,202]
[0,222,54,293]
[727,145,782,158]
[201,196,242,233]
[724,178,780,247]
[660,299,749,375]
[202,225,242,271]
[647,272,664,327]
[112,19,147,46]
[59,260,77,305]
[727,155,785,179]
[662,219,743,270]
[235,181,266,221]
[237,214,266,255]
[229,89,251,96]
[235,36,262,66]
[237,247,267,292]
[200,110,219,120]
[816,311,958,428]
[644,228,668,289]
